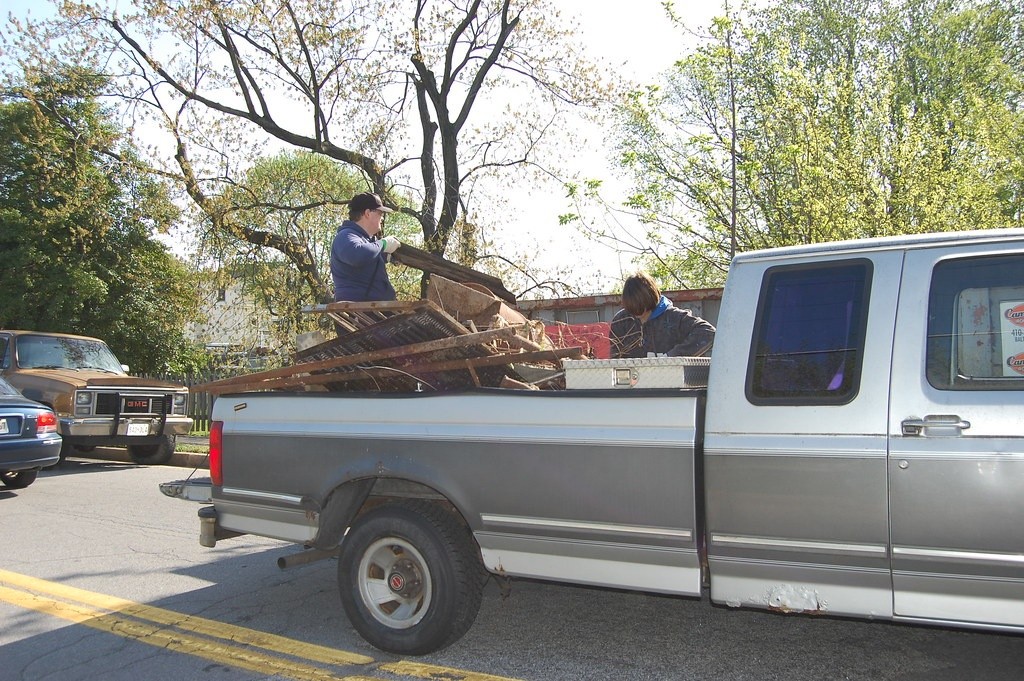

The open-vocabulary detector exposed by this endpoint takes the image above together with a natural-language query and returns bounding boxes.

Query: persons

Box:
[329,191,401,337]
[608,271,716,359]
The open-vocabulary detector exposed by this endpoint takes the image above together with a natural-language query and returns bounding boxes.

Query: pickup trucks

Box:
[198,229,1024,658]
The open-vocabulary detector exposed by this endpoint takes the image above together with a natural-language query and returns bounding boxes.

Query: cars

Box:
[0,376,63,489]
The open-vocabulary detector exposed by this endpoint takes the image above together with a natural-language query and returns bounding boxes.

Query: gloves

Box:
[380,236,402,255]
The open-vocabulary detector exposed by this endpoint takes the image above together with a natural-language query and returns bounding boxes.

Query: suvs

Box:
[0,330,194,465]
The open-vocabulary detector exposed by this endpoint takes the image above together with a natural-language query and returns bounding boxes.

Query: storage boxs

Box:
[563,356,711,388]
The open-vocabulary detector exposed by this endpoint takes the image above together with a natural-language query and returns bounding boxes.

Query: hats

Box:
[346,192,394,213]
